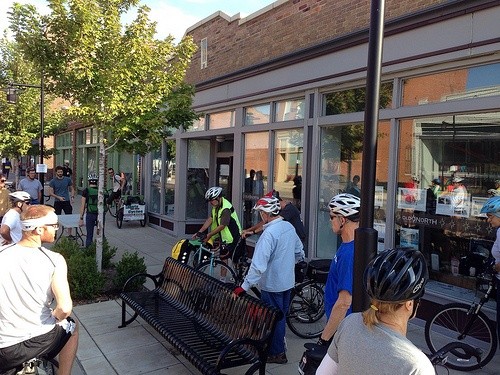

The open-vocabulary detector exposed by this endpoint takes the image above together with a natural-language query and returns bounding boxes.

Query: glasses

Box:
[109,172,113,174]
[329,214,349,220]
[0,180,6,183]
[45,223,59,230]
[25,201,30,205]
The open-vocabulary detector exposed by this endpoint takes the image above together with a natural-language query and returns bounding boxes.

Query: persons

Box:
[232,191,302,364]
[427,176,470,215]
[17,167,43,204]
[347,176,361,197]
[106,168,126,209]
[479,195,500,313]
[0,191,79,375]
[80,174,109,248]
[315,246,435,375]
[3,158,12,179]
[298,193,361,375]
[245,169,264,226]
[0,173,12,224]
[292,175,302,210]
[61,163,72,177]
[192,186,246,283]
[48,166,75,237]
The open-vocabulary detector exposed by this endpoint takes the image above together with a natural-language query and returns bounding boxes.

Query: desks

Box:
[54,215,87,246]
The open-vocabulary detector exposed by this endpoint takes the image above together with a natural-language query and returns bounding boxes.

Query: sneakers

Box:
[266,351,288,364]
[68,235,75,240]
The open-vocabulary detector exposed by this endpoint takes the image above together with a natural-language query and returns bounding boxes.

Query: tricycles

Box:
[105,188,147,230]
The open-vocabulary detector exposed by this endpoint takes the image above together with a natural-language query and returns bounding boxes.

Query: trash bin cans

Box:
[20,169,26,176]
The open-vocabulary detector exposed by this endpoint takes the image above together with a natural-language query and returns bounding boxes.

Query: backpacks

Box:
[85,186,109,213]
[194,238,214,265]
[172,239,191,264]
[114,172,127,187]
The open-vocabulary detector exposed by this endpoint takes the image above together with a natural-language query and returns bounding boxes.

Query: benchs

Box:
[118,257,285,375]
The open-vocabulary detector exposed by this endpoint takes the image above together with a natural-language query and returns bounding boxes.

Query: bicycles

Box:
[9,357,59,375]
[425,259,497,372]
[188,231,333,339]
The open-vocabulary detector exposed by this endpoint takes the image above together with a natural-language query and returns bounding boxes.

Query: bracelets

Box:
[71,196,74,198]
[253,230,256,234]
[80,217,83,220]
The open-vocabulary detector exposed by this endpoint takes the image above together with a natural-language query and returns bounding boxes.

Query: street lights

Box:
[0,77,44,204]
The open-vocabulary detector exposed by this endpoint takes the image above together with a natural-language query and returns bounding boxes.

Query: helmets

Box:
[205,187,223,201]
[327,193,361,221]
[87,173,99,182]
[253,196,281,214]
[363,247,429,302]
[481,196,500,218]
[8,191,30,206]
